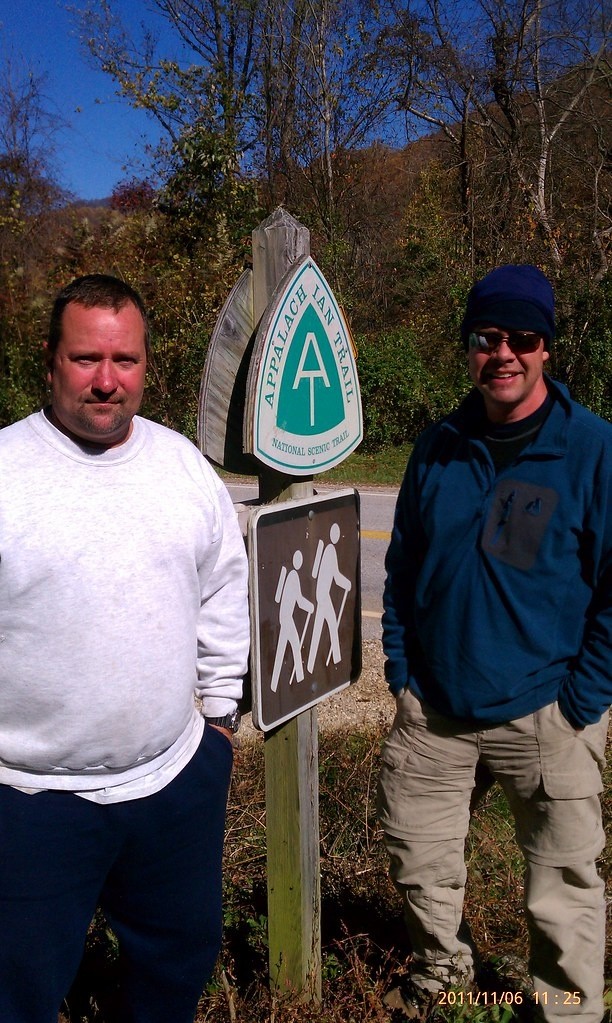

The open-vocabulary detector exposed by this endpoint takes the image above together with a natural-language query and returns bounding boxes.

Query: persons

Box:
[377,265,612,1023]
[0,272,251,1023]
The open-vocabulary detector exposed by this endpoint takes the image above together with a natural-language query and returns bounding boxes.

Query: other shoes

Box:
[381,958,475,1023]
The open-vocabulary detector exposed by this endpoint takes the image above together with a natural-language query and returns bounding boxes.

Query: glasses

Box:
[468,332,542,354]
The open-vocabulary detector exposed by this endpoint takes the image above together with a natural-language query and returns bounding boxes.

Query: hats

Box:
[460,264,554,353]
